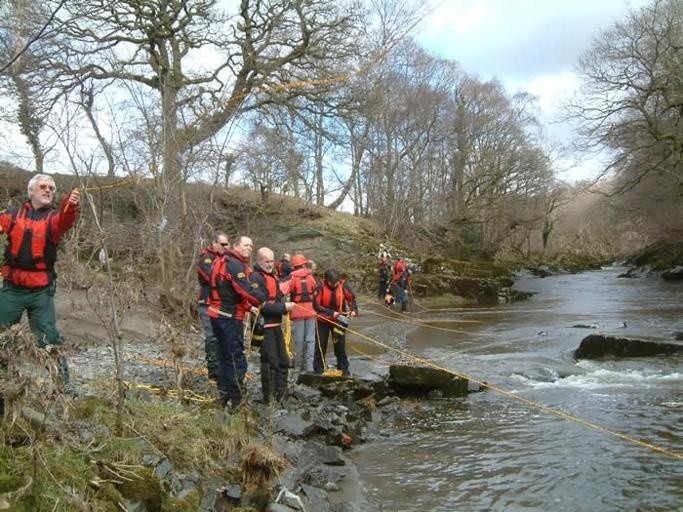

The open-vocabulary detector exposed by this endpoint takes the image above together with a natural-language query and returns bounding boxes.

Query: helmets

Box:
[291,254,307,266]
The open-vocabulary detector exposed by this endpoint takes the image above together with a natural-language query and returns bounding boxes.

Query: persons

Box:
[396,268,413,315]
[0,170,83,400]
[392,258,405,281]
[378,256,391,302]
[194,229,359,415]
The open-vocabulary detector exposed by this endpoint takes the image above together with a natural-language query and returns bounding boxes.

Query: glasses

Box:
[216,241,229,246]
[36,183,56,191]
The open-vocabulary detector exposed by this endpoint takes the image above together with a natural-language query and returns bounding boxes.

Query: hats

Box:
[324,269,340,283]
